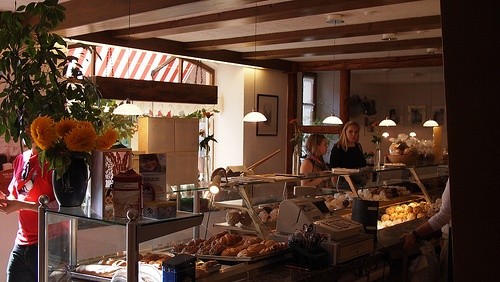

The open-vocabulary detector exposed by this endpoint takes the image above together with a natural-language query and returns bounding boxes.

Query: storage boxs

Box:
[111,117,199,221]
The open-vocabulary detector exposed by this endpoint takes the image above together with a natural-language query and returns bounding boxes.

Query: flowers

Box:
[370,120,384,143]
[31,115,119,181]
[288,118,306,145]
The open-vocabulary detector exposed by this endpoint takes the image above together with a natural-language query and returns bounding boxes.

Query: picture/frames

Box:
[407,105,427,126]
[256,94,279,137]
[431,106,446,127]
[387,105,403,131]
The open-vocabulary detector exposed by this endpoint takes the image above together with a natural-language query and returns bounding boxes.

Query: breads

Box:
[171,230,285,258]
[226,181,420,226]
[75,251,219,279]
[380,198,442,221]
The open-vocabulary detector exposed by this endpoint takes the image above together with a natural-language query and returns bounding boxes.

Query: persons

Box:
[330,120,366,190]
[397,176,451,282]
[0,118,70,282]
[300,133,337,190]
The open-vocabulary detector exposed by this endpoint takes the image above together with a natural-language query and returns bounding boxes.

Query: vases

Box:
[292,145,299,175]
[52,151,88,207]
[375,141,380,168]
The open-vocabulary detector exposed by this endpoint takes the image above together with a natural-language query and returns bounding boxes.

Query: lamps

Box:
[378,33,397,126]
[243,0,267,122]
[322,14,344,124]
[422,47,439,126]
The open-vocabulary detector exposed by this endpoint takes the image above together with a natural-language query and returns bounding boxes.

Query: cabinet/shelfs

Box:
[212,159,449,234]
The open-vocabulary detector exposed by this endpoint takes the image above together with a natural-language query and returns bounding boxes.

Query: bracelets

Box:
[412,229,418,238]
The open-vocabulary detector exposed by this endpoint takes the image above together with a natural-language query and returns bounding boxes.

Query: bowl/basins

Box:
[387,154,417,166]
